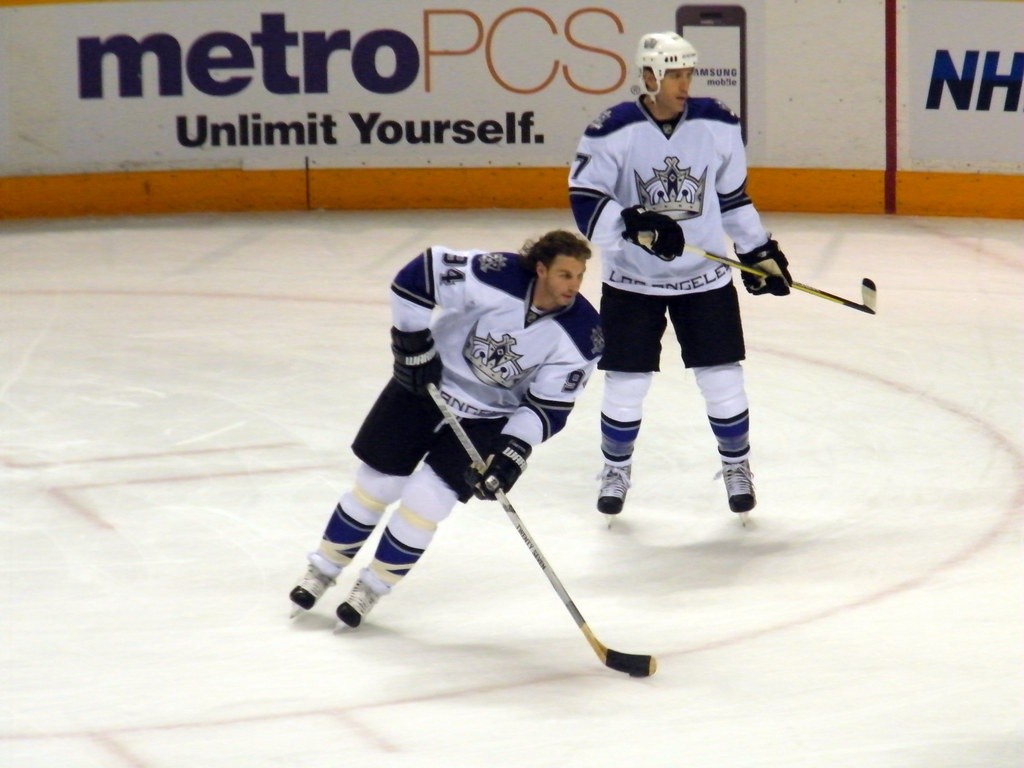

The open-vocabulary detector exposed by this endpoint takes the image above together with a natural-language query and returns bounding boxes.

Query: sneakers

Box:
[288,564,337,619]
[714,461,756,526]
[594,463,632,529]
[334,580,380,634]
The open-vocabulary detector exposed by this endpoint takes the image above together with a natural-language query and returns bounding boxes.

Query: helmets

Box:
[636,31,697,104]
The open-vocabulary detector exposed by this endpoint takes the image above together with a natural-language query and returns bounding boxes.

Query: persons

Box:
[568,31,793,515]
[289,231,606,628]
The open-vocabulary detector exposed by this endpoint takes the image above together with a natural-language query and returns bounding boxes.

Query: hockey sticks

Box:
[427,383,657,680]
[636,232,878,315]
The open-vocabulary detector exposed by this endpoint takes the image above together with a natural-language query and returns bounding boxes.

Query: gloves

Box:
[465,444,528,501]
[620,205,685,261]
[391,338,444,395]
[734,239,793,296]
